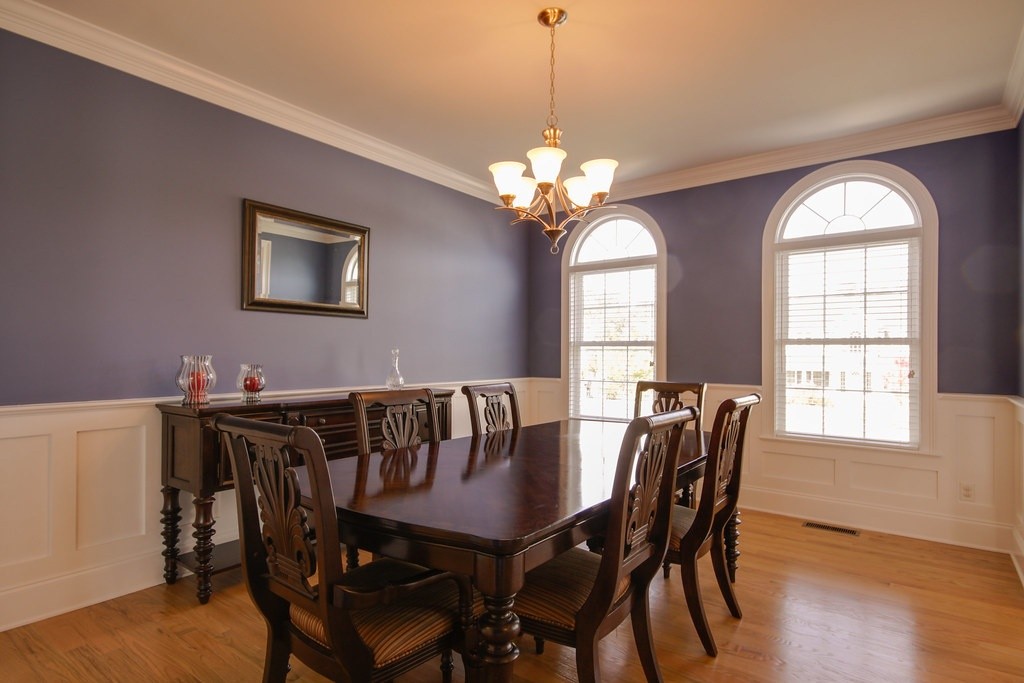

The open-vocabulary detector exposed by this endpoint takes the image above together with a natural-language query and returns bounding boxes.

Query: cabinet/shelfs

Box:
[154,386,455,606]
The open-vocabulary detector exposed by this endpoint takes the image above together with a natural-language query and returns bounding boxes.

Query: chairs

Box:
[346,388,442,572]
[586,392,763,656]
[634,380,708,579]
[461,381,522,437]
[511,406,701,683]
[209,412,487,683]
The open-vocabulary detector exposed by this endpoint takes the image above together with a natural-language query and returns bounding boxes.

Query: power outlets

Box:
[960,481,975,503]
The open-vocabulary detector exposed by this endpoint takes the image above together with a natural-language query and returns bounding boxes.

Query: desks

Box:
[291,418,743,683]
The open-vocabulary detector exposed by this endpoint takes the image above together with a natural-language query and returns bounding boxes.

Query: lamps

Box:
[487,6,618,255]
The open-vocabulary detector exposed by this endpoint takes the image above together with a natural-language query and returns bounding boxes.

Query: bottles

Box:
[174,354,217,404]
[237,362,265,403]
[385,347,405,389]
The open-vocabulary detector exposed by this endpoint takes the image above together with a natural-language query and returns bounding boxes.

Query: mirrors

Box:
[240,198,370,320]
